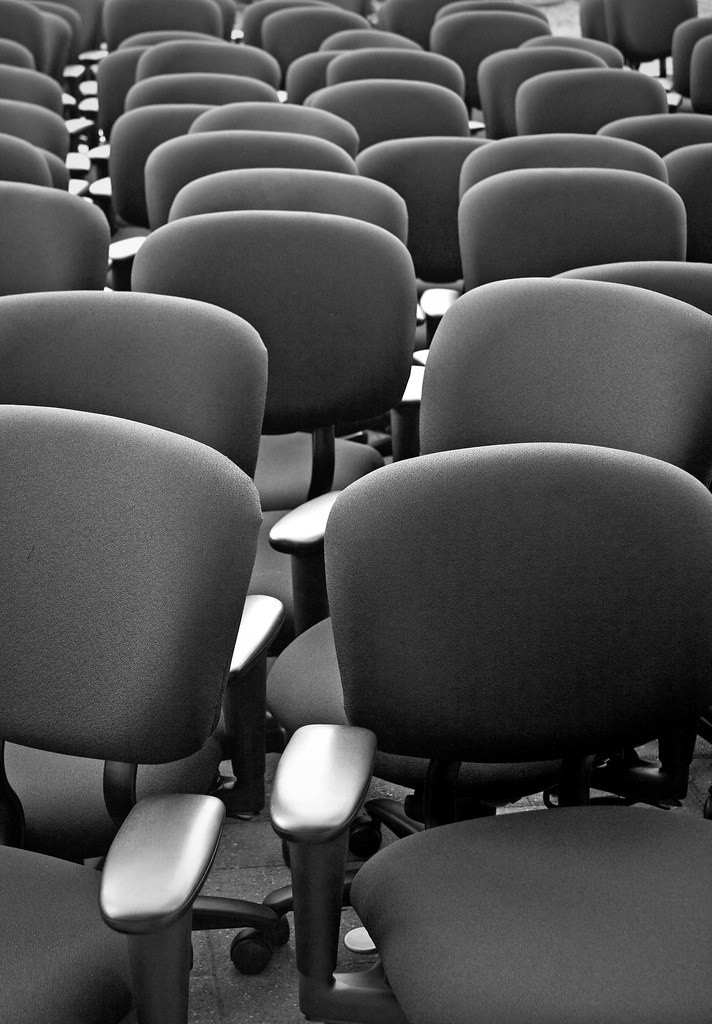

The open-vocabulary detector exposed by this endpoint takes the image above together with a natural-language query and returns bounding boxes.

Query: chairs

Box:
[0,0,712,1024]
